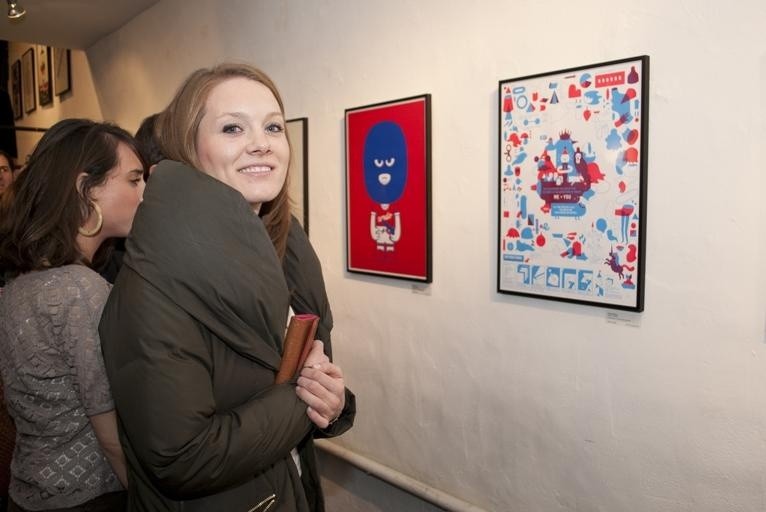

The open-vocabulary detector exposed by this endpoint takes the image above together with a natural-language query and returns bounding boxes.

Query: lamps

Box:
[7,0,26,19]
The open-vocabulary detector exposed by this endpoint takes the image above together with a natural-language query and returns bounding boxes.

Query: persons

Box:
[0,150,16,197]
[362,119,408,253]
[0,117,149,512]
[104,109,334,364]
[98,61,356,512]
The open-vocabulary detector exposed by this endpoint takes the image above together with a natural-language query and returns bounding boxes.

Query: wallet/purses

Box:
[275,313,321,388]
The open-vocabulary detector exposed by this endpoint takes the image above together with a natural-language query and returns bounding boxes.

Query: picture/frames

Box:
[11,44,71,120]
[344,94,432,283]
[496,55,649,313]
[286,117,308,239]
